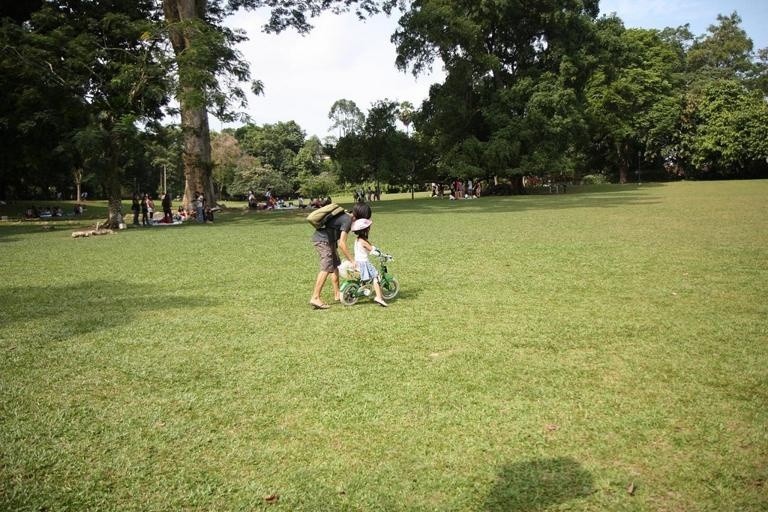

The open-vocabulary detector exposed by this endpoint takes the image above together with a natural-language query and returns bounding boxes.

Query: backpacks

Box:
[306,203,344,229]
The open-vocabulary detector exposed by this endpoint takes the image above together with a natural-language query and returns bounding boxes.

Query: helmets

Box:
[351,218,372,232]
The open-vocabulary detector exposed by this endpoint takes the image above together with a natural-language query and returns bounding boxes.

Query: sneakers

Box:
[374,297,387,307]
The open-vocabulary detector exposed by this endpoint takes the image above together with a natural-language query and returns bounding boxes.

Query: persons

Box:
[132,192,212,226]
[27,184,88,218]
[353,187,379,204]
[248,188,332,211]
[309,204,371,310]
[493,170,567,196]
[426,177,481,200]
[352,218,391,307]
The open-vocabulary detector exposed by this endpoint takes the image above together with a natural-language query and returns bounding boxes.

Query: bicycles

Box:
[338,255,401,306]
